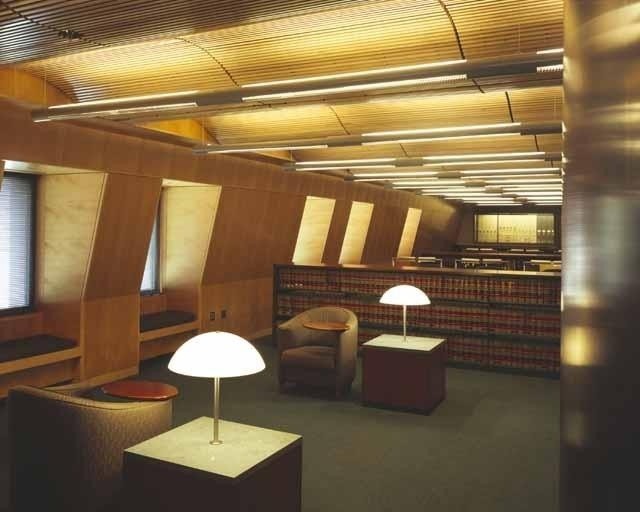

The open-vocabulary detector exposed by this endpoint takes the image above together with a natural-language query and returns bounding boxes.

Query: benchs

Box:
[140,310,199,363]
[0,334,80,399]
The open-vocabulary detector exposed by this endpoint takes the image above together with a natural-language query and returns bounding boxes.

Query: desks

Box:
[303,321,348,332]
[101,380,179,400]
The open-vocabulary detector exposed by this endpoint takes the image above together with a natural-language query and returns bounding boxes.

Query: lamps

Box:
[167,329,266,444]
[379,284,431,342]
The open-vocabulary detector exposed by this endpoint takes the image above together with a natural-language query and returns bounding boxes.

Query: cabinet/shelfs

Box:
[362,332,448,416]
[123,415,303,512]
[272,242,562,380]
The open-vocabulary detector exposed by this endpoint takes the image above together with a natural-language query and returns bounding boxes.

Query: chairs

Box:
[276,306,358,400]
[7,384,173,512]
